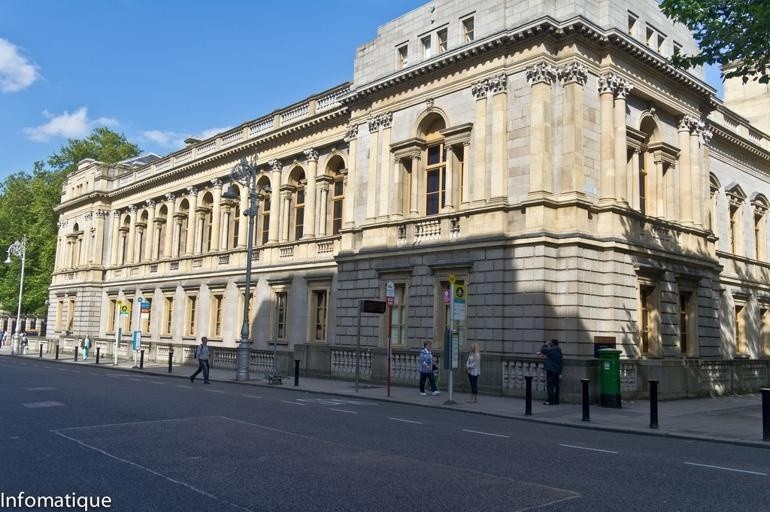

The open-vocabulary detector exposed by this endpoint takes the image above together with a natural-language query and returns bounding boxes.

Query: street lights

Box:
[222,157,261,382]
[3,234,29,358]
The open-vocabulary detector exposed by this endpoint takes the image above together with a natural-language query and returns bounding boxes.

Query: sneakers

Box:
[431,390,441,396]
[418,392,430,396]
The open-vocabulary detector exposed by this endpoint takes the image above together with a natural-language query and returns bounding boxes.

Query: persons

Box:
[417,341,441,396]
[20,333,28,355]
[0,330,4,349]
[80,334,92,360]
[189,336,210,384]
[2,330,7,346]
[541,338,563,406]
[465,342,481,404]
